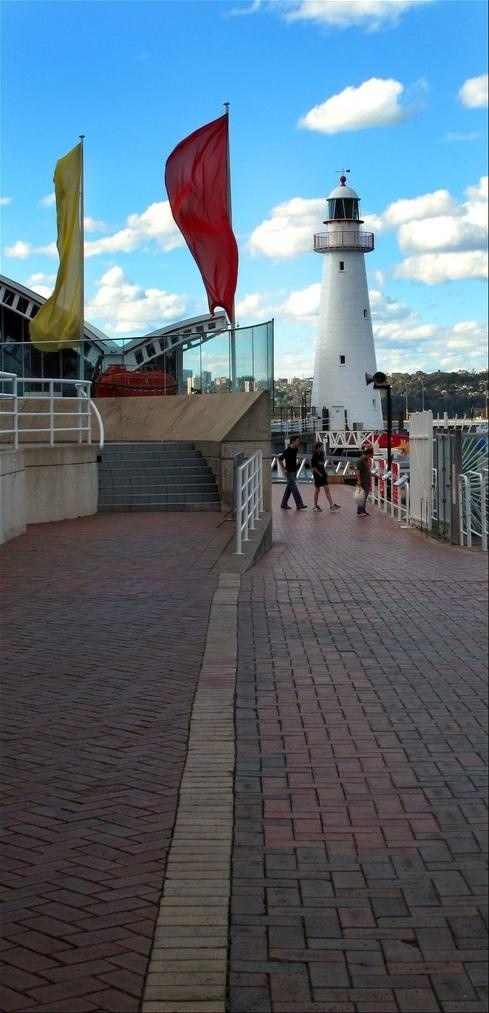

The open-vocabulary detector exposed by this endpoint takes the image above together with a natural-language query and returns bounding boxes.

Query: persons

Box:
[352,445,374,516]
[310,442,342,512]
[278,434,308,510]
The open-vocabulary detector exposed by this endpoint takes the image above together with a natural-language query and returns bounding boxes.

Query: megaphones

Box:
[373,372,387,386]
[365,372,374,385]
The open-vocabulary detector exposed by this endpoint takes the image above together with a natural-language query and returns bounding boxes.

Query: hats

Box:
[363,447,373,454]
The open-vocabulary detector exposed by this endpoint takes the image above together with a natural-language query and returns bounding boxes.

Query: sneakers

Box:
[329,504,340,511]
[356,511,366,516]
[313,505,323,511]
[364,512,371,515]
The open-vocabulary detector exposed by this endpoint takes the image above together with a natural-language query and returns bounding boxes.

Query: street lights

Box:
[405,382,410,420]
[477,381,488,418]
[419,378,424,411]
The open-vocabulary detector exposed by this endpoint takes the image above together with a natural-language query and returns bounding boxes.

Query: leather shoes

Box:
[297,504,308,509]
[280,505,291,509]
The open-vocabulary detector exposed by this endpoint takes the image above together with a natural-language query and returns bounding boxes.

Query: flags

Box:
[163,105,240,318]
[26,138,82,352]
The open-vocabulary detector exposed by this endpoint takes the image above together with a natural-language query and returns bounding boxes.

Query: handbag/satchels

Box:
[354,485,365,506]
